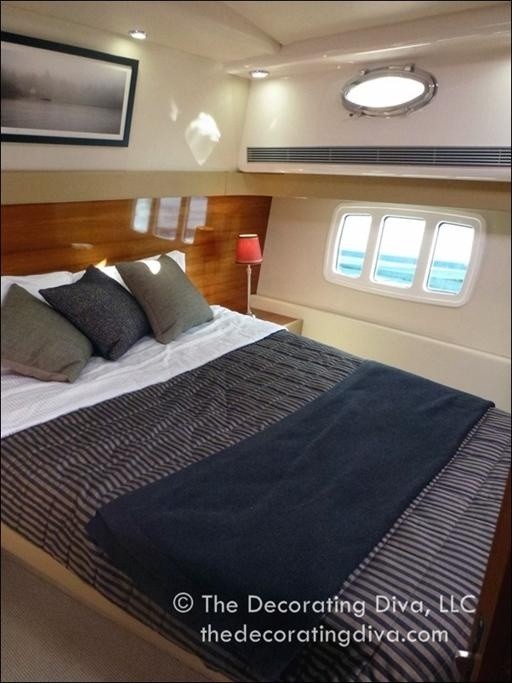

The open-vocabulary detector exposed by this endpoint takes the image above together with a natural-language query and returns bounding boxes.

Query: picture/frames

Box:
[0,31,139,147]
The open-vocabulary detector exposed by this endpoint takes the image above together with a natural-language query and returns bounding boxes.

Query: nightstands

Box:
[236,307,303,336]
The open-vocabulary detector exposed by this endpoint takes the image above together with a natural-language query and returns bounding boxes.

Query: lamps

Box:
[235,233,263,318]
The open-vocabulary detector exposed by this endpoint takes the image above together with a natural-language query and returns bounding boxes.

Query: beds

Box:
[1,195,511,682]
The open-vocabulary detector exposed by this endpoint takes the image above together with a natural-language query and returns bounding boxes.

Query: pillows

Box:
[1,249,214,383]
